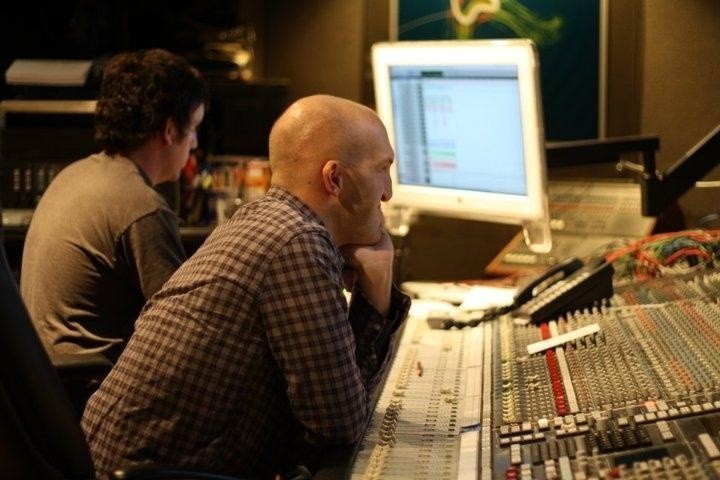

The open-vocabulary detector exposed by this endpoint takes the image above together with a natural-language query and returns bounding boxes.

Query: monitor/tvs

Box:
[369,39,549,226]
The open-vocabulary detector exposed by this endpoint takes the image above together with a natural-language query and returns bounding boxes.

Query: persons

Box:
[84,94,394,480]
[22,48,206,407]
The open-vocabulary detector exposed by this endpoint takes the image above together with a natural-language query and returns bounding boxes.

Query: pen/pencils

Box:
[178,168,243,228]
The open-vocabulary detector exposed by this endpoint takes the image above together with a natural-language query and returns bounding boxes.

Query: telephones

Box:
[443,255,614,330]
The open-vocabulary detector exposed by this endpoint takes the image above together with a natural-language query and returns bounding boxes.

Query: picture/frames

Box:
[364,0,643,164]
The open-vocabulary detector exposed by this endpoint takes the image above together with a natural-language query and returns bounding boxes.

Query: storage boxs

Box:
[201,62,290,157]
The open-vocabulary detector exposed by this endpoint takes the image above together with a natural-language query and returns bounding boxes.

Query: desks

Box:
[2,208,409,239]
[342,275,720,480]
